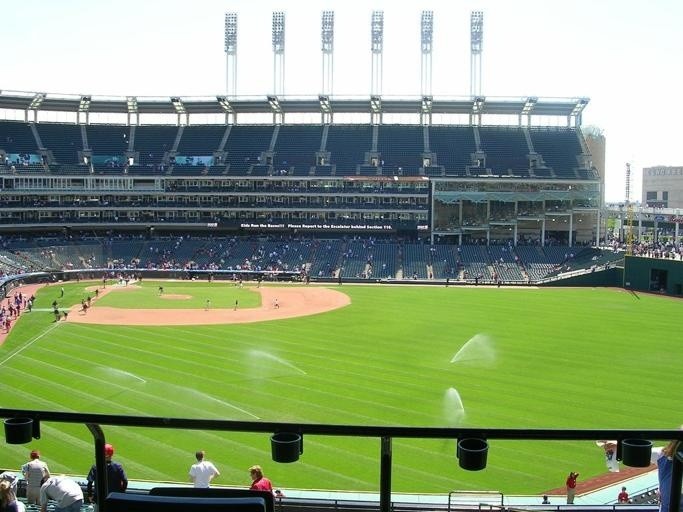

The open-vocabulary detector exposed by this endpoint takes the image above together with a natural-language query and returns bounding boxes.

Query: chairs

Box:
[100,492,265,511]
[148,487,275,512]
[0,497,96,512]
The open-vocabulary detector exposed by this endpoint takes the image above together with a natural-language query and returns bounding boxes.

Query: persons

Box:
[603,425,683,512]
[248,464,287,497]
[0,444,128,512]
[0,152,683,333]
[188,451,220,489]
[565,472,579,504]
[542,495,550,504]
